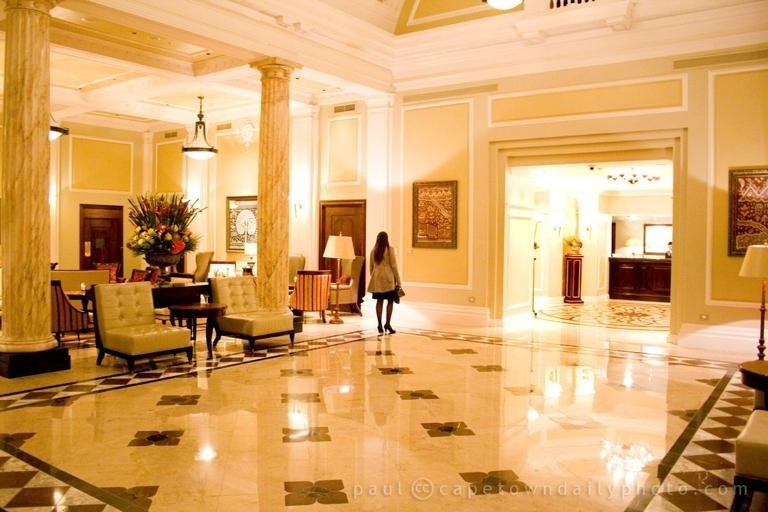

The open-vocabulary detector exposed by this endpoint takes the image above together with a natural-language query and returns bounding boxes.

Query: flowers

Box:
[126,191,208,257]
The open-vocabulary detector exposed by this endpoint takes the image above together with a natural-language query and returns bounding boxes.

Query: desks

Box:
[63,290,89,311]
[169,304,227,359]
[738,361,768,409]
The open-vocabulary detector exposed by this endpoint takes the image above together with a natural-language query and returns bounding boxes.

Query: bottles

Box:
[80,283,85,290]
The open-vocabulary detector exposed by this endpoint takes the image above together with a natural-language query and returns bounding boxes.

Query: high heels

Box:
[384,324,395,334]
[378,324,384,333]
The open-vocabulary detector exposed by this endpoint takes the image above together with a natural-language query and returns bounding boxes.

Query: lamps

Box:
[323,231,356,324]
[49,111,68,141]
[182,95,218,161]
[739,243,768,361]
[606,166,661,184]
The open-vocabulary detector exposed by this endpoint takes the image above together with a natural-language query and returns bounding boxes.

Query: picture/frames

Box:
[412,181,457,249]
[225,196,258,253]
[727,165,768,257]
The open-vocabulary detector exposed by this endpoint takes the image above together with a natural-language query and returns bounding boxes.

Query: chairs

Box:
[91,281,193,371]
[51,252,214,346]
[289,270,332,323]
[207,277,295,353]
[289,256,305,295]
[328,256,364,317]
[730,409,768,512]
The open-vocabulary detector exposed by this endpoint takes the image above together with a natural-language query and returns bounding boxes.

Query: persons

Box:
[664,242,672,259]
[368,231,404,334]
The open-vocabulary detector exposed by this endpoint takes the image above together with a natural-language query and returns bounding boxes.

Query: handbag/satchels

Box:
[394,286,399,304]
[398,288,405,297]
